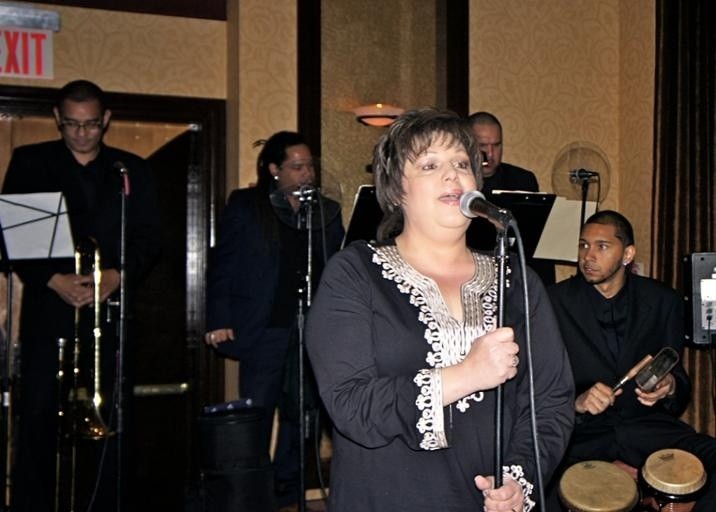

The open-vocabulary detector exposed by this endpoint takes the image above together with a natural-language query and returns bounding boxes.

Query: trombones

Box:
[56,235,116,511]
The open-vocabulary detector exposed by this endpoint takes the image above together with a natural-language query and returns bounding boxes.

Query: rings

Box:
[511,353,518,367]
[210,333,215,342]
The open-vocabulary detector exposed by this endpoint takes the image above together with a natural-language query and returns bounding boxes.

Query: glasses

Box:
[59,116,104,131]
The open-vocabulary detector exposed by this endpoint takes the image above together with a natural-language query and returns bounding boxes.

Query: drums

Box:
[558,460,640,512]
[637,448,711,511]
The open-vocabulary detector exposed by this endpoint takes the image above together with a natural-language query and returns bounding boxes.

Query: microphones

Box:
[459,189,513,233]
[288,184,323,202]
[567,168,600,182]
[116,160,132,198]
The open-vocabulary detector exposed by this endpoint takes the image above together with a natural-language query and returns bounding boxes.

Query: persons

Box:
[0,77,161,512]
[204,130,347,511]
[546,209,716,512]
[465,109,559,287]
[302,102,576,512]
